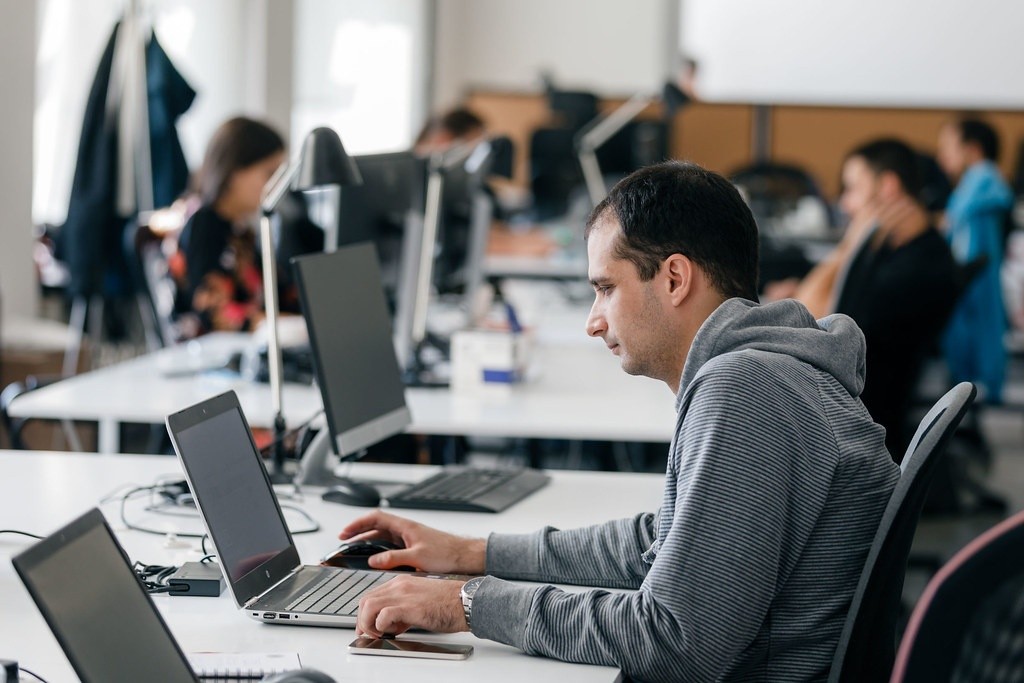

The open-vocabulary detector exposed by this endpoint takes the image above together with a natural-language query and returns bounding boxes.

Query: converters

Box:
[167,561,228,597]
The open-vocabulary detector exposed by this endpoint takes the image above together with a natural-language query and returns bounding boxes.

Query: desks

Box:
[1,436,678,683]
[6,287,685,454]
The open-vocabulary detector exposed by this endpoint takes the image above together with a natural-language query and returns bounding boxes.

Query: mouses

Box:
[319,540,416,572]
[261,667,335,683]
[321,481,381,507]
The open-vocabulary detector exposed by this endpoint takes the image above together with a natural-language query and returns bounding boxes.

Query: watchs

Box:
[459,577,484,631]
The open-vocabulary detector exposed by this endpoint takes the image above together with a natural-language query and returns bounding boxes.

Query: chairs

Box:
[3,135,1024,683]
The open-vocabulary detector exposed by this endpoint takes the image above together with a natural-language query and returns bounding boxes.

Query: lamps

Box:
[260,127,365,486]
[579,79,697,205]
[390,136,519,385]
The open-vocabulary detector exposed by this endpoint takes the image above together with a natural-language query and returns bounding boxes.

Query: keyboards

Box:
[386,469,551,513]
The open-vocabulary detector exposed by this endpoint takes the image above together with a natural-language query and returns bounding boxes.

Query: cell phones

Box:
[346,635,474,660]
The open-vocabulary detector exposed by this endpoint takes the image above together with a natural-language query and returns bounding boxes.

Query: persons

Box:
[162,56,700,346]
[796,114,953,405]
[937,109,1009,263]
[339,162,903,683]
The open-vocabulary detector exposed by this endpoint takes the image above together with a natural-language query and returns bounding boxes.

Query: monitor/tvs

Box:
[289,150,474,495]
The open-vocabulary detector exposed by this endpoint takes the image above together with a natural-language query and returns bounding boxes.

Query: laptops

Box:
[165,389,476,632]
[12,507,302,683]
